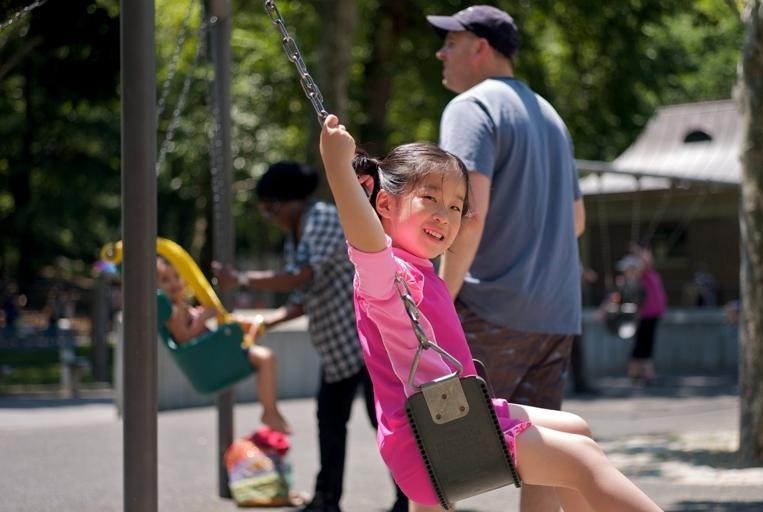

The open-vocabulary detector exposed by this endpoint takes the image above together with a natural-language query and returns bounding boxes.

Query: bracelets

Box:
[256,313,264,335]
[238,271,249,290]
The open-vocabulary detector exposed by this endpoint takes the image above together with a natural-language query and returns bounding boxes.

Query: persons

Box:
[205,157,418,512]
[156,256,295,436]
[319,108,668,512]
[623,242,668,385]
[411,6,590,411]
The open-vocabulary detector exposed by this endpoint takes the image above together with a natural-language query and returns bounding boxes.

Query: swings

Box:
[156,0,262,392]
[592,165,642,340]
[265,0,523,512]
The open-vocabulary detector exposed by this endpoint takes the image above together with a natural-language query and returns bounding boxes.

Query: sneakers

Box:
[290,497,341,512]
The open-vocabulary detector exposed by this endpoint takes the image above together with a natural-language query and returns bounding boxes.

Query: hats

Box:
[425,6,520,55]
[255,160,323,203]
